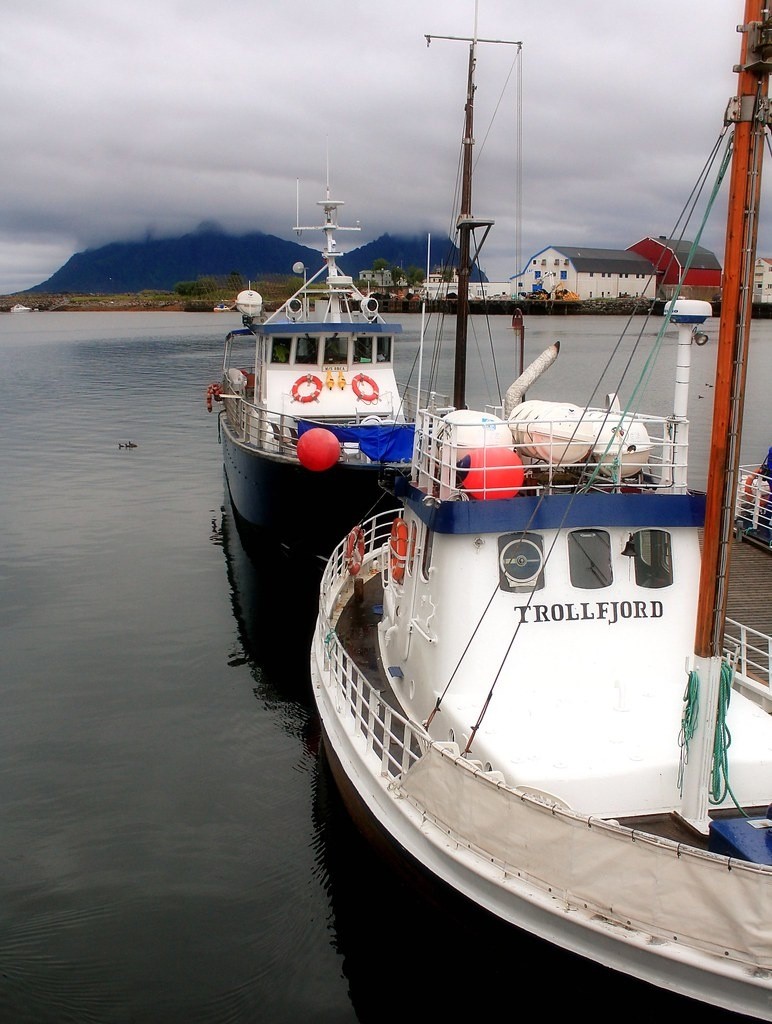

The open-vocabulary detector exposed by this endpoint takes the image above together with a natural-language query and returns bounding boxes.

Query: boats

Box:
[213,299,232,313]
[217,132,433,540]
[9,303,33,314]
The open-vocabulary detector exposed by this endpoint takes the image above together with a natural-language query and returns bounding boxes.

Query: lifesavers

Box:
[390,518,408,579]
[291,375,321,402]
[745,469,770,506]
[352,373,378,401]
[347,526,365,573]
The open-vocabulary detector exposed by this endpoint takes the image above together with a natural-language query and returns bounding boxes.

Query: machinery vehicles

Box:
[526,281,580,303]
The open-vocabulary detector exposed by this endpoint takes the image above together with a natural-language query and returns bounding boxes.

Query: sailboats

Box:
[310,0,772,1024]
